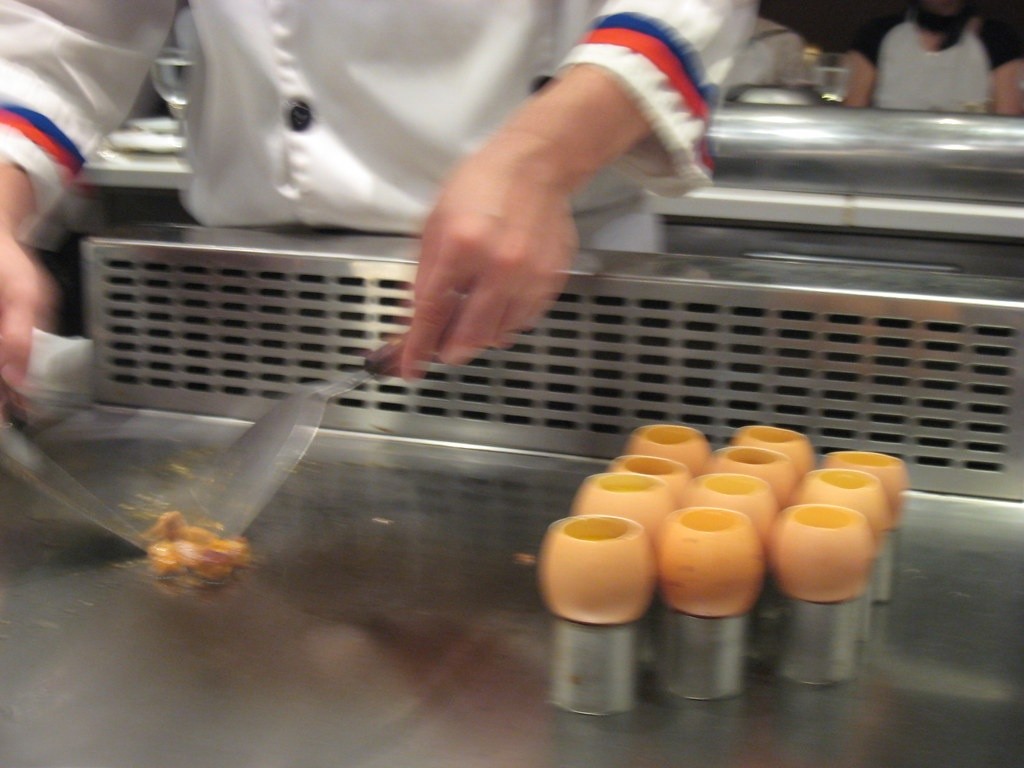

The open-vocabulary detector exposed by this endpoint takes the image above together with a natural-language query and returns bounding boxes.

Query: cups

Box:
[802,52,851,100]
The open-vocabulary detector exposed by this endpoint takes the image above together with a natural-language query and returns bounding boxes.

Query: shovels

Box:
[185,337,406,540]
[1,376,152,554]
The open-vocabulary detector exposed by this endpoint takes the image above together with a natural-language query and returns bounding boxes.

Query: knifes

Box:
[0,403,153,553]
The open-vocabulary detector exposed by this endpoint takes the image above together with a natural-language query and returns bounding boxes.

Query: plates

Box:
[107,130,186,152]
[127,115,178,133]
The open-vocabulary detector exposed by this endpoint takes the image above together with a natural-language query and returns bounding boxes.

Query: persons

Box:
[1,0,767,424]
[731,0,1024,114]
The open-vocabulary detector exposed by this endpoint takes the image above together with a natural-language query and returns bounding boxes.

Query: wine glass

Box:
[150,48,192,136]
